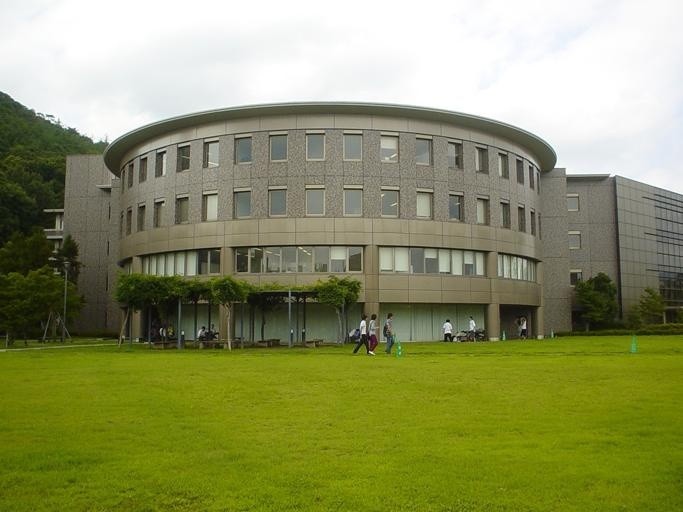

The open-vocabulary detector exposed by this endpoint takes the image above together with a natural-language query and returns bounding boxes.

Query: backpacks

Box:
[383,323,388,337]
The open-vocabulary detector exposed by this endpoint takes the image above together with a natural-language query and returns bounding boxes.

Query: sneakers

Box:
[368,350,375,355]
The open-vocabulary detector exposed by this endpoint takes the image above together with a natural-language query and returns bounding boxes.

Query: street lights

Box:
[62,261,71,342]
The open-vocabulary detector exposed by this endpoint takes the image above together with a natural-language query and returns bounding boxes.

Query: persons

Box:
[353,314,380,356]
[515,317,527,341]
[442,318,452,342]
[383,313,394,354]
[198,324,216,341]
[159,323,174,337]
[468,316,476,343]
